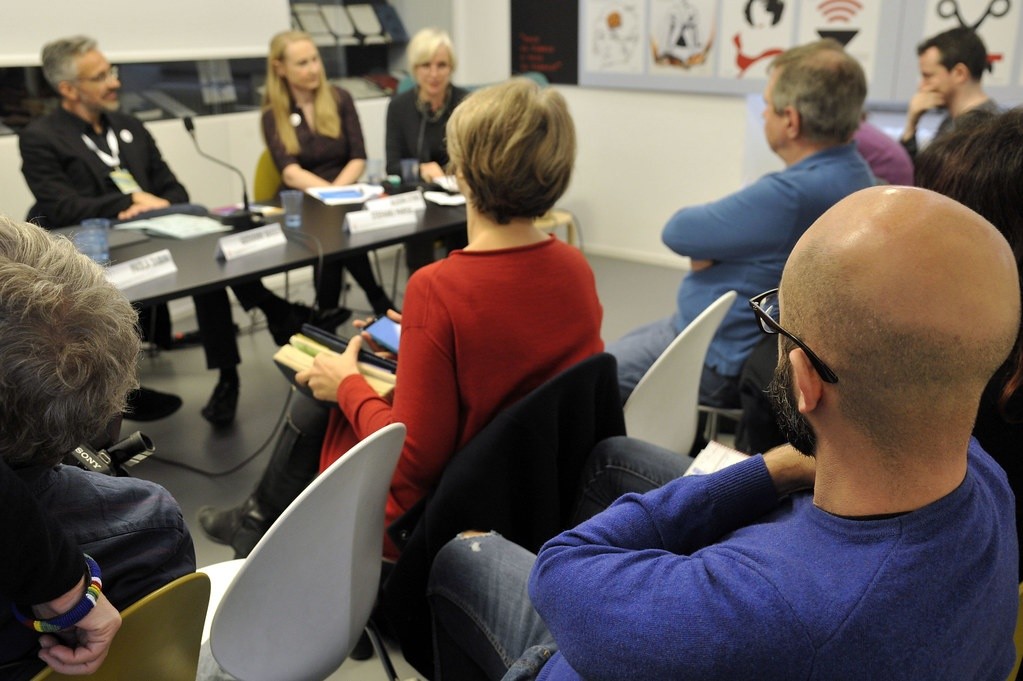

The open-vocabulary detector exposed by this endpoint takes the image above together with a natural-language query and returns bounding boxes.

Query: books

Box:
[274,324,396,410]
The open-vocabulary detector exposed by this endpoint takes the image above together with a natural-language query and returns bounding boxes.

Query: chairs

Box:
[363,347,634,681]
[246,145,396,320]
[195,420,406,681]
[622,289,738,458]
[31,570,213,681]
[22,197,166,378]
[699,405,745,458]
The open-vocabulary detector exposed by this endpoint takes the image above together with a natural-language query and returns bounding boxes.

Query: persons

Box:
[199,76,602,658]
[427,186,1023,681]
[258,31,402,327]
[18,35,352,427]
[0,215,196,680]
[0,455,122,676]
[385,28,469,279]
[607,26,1022,457]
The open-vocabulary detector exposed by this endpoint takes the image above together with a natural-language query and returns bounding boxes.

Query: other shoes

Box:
[269,303,352,347]
[199,383,238,435]
[119,385,184,422]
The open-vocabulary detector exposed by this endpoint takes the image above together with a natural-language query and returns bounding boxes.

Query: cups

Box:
[281,192,303,227]
[82,219,109,264]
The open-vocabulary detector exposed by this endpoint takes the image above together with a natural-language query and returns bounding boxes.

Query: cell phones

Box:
[361,314,402,353]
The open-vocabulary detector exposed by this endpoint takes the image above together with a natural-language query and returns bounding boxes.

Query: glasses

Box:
[72,66,120,85]
[749,290,838,387]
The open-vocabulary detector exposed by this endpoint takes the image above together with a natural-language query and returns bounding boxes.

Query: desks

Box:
[41,173,471,304]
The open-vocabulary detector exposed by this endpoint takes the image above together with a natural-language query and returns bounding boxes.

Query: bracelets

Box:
[20,549,110,632]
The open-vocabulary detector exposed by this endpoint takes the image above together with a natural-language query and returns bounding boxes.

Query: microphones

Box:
[183,114,265,230]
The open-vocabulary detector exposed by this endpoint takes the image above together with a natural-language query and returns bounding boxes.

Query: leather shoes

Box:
[198,501,265,561]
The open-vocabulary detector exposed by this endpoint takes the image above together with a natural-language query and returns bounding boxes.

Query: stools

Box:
[535,211,576,249]
